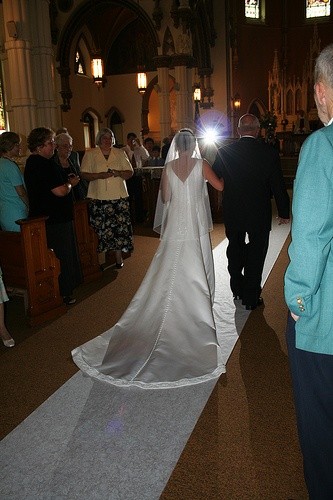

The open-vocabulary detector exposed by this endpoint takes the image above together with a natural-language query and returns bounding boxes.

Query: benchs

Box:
[0,200,103,330]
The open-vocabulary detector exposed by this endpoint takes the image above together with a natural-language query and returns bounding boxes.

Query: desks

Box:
[127,166,163,238]
[277,131,311,157]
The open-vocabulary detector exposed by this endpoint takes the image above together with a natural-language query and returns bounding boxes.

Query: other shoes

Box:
[233,290,243,299]
[2,338,15,347]
[245,297,264,310]
[116,261,124,269]
[65,295,77,304]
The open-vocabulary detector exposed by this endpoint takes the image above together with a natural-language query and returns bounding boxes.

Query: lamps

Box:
[92,55,105,91]
[193,82,202,102]
[233,92,241,112]
[137,64,147,93]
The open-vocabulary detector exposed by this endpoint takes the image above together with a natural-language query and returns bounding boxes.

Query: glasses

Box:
[58,144,73,149]
[43,140,57,146]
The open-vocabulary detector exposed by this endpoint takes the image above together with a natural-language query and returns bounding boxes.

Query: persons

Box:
[81,127,134,269]
[120,133,175,224]
[160,128,224,306]
[49,128,88,202]
[0,267,15,347]
[284,42,333,500]
[211,113,289,310]
[0,132,30,232]
[24,127,80,305]
